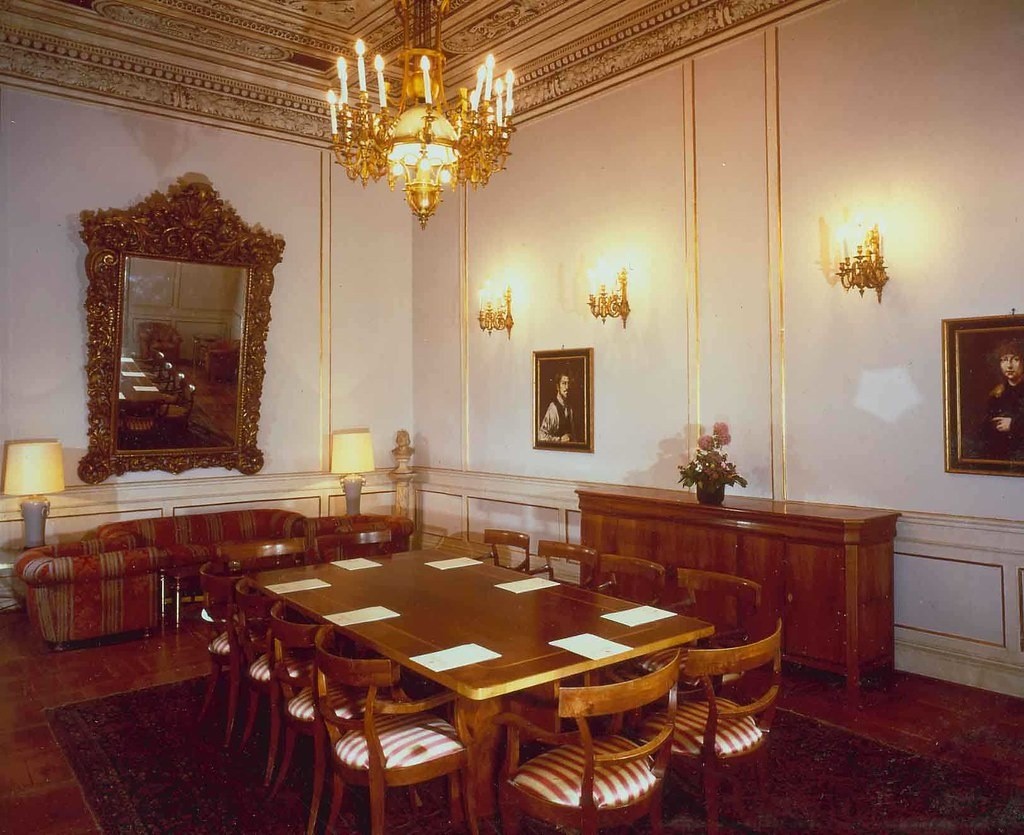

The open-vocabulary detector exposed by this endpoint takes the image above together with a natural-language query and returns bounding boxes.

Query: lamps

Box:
[328,0,514,231]
[586,258,631,331]
[477,277,514,341]
[330,432,375,515]
[836,216,889,305]
[4,443,65,550]
[532,346,595,455]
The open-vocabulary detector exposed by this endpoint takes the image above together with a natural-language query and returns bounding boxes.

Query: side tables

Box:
[0,551,26,609]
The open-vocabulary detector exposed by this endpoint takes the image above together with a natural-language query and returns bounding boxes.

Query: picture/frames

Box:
[940,314,1024,478]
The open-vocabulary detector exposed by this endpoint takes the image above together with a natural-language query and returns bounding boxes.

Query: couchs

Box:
[138,321,181,364]
[13,508,413,652]
[191,333,223,367]
[202,339,239,382]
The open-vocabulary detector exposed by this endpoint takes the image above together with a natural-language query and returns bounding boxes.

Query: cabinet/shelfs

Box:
[574,486,903,707]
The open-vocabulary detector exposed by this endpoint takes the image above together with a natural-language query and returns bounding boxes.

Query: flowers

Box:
[677,421,748,490]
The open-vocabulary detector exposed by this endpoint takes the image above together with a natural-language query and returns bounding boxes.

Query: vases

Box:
[697,484,725,504]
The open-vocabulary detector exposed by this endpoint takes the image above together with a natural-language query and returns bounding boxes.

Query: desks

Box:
[260,549,715,835]
[119,356,164,417]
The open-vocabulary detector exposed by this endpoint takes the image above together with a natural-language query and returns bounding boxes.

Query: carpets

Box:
[117,372,234,450]
[41,669,1024,835]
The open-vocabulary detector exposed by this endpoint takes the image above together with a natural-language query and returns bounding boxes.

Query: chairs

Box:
[306,627,481,835]
[140,351,164,379]
[476,529,530,573]
[160,373,187,415]
[597,553,665,607]
[120,408,159,450]
[151,363,176,389]
[526,541,597,591]
[271,603,393,835]
[159,384,197,439]
[236,577,314,798]
[636,617,782,834]
[606,567,761,726]
[491,647,682,835]
[195,562,271,749]
[161,558,290,630]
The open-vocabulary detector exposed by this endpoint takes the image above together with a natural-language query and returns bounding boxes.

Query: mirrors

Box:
[77,180,285,486]
